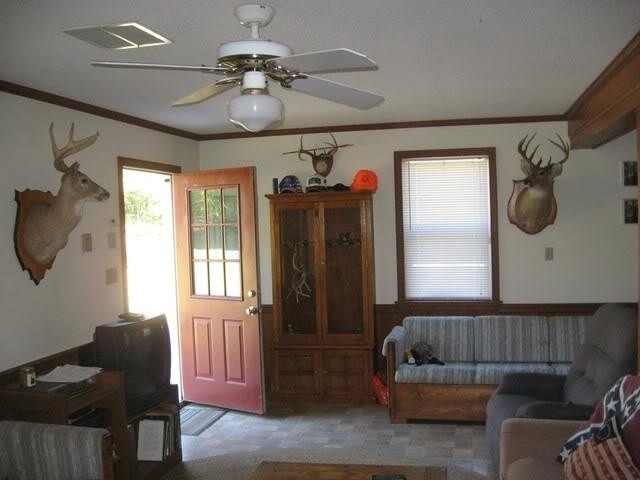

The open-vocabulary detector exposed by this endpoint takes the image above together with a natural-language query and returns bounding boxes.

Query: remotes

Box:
[118,313,143,321]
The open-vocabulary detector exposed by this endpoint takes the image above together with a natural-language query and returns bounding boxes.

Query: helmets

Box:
[280,176,303,194]
[306,176,327,192]
[350,170,377,192]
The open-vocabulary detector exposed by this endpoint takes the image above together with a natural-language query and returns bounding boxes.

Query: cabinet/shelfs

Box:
[264,189,376,401]
[2,368,182,480]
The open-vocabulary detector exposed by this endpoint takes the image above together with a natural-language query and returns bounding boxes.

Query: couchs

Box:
[382,315,594,426]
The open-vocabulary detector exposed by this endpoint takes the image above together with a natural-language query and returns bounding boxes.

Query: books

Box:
[136,402,180,464]
[37,362,106,424]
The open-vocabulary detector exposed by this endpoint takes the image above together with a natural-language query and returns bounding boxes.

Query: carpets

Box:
[178,408,230,437]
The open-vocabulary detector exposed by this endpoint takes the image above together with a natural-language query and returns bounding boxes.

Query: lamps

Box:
[227,69,284,134]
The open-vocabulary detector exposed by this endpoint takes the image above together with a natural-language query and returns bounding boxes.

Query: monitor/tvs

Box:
[78,314,173,418]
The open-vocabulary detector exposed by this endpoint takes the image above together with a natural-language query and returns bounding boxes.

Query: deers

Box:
[515,130,569,229]
[298,131,338,177]
[22,121,110,265]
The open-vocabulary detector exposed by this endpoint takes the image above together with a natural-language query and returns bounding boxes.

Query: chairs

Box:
[484,304,639,480]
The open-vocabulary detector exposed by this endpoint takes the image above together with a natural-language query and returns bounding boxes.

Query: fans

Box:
[88,3,387,111]
